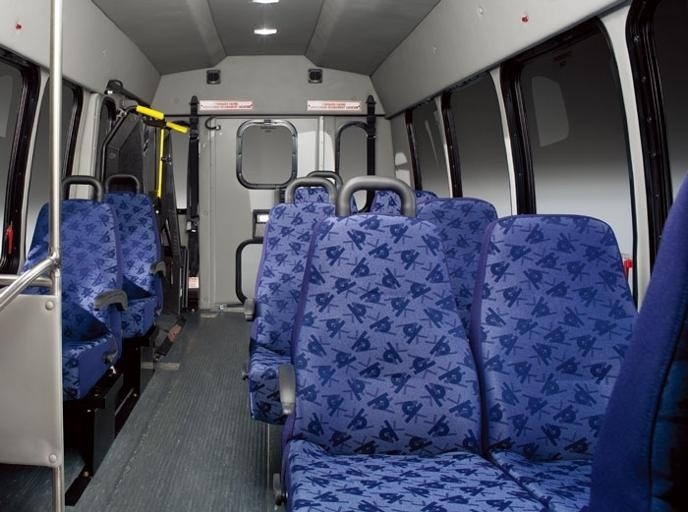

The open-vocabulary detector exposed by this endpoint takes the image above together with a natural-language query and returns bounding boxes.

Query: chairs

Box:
[245,176,366,510]
[276,177,546,512]
[476,214,643,512]
[16,174,128,476]
[286,170,499,343]
[101,172,168,399]
